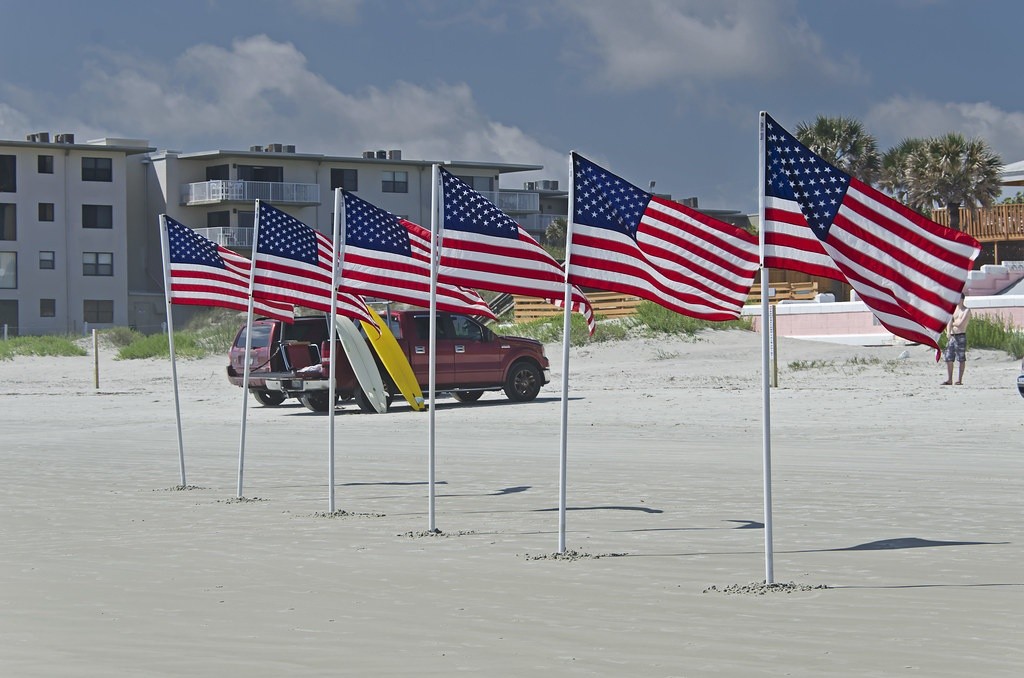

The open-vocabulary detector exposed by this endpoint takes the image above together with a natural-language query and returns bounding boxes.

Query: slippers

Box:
[956,382,961,385]
[941,382,952,385]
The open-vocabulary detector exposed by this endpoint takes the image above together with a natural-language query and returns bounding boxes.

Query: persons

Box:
[942,293,970,385]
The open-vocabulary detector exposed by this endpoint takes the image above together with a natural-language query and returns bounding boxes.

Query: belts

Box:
[950,333,965,336]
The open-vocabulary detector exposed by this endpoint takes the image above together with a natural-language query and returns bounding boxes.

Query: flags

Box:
[252,199,382,342]
[163,214,295,325]
[337,189,500,323]
[437,164,596,337]
[763,115,983,363]
[568,152,760,322]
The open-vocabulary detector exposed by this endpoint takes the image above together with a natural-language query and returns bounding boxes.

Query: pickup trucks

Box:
[265,309,550,415]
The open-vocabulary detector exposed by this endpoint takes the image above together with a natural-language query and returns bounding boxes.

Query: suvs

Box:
[226,315,355,412]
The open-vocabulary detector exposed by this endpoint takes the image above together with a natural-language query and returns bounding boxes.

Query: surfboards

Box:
[335,314,387,414]
[359,305,426,412]
